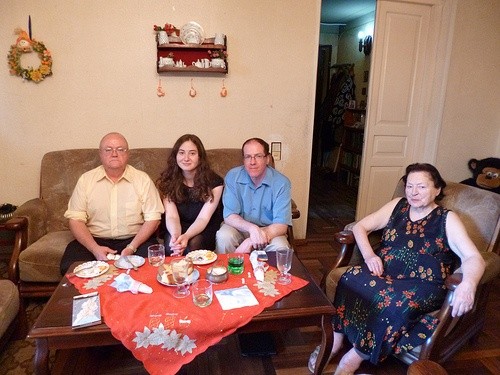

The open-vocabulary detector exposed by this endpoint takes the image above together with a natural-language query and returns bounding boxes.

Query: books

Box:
[72,292,101,329]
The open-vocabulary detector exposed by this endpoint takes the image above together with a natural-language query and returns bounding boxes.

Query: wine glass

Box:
[276,247,294,286]
[170,257,190,298]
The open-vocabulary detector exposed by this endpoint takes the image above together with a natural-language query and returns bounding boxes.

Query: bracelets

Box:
[127,245,137,252]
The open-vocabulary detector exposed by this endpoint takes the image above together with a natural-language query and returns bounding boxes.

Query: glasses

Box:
[100,147,128,155]
[243,153,268,162]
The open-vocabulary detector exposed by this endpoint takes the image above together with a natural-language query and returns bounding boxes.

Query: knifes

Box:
[124,256,138,270]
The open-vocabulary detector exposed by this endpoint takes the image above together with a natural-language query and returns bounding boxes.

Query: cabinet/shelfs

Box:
[339,108,366,186]
[156,34,229,74]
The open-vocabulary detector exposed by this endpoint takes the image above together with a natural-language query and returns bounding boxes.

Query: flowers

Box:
[6,29,53,83]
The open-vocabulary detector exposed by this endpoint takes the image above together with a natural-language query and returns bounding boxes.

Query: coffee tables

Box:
[26,251,337,375]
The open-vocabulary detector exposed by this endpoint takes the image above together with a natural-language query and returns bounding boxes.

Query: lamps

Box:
[357,31,372,57]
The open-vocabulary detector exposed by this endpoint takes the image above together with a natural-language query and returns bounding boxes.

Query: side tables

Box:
[0,217,16,278]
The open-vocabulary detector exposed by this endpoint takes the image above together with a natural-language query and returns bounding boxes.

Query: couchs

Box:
[0,279,26,352]
[5,148,300,297]
[319,175,500,368]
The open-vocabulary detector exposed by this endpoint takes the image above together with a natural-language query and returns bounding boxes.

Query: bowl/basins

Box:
[207,266,228,283]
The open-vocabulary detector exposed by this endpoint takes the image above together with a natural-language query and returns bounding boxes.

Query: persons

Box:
[155,134,224,256]
[60,133,165,278]
[308,162,487,375]
[216,138,293,254]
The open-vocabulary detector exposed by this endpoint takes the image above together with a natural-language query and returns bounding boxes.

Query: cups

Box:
[192,279,213,308]
[226,252,245,274]
[147,244,165,267]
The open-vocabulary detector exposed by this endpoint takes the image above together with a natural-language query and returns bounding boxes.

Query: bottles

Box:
[214,32,224,46]
[158,57,164,67]
[175,58,211,68]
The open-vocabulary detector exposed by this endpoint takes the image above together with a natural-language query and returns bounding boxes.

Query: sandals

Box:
[308,344,333,373]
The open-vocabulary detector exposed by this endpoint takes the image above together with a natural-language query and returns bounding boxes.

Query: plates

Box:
[157,268,199,286]
[180,22,205,45]
[187,250,218,265]
[73,261,110,278]
[114,255,145,270]
